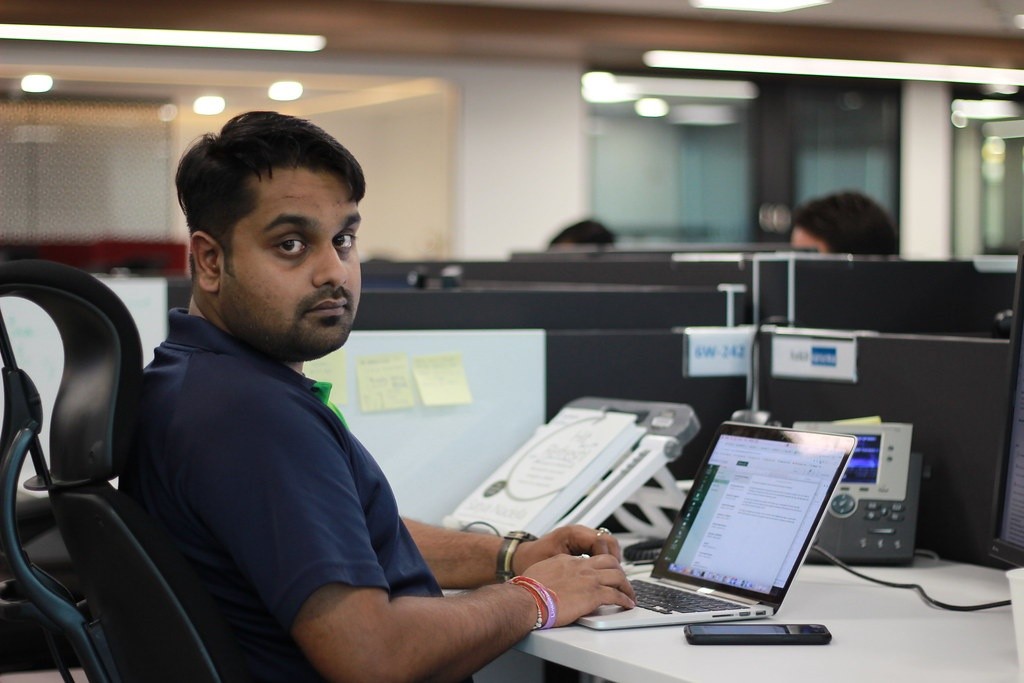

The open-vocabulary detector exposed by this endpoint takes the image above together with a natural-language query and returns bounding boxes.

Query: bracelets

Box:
[496,530,541,582]
[506,575,558,630]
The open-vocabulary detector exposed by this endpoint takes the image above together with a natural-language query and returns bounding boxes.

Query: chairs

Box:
[0,260,329,683]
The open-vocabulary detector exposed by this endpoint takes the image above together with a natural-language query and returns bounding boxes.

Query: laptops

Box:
[574,421,858,631]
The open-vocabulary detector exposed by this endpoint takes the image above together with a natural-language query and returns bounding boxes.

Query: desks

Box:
[441,532,1024,683]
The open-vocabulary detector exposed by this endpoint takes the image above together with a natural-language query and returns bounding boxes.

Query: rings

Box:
[582,553,590,559]
[596,527,612,537]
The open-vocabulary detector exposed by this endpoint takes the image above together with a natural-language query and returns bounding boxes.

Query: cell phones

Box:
[683,625,832,646]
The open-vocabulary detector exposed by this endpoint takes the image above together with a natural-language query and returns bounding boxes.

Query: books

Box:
[454,407,647,539]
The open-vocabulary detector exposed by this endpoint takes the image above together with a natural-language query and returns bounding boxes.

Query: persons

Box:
[131,106,636,683]
[548,220,615,253]
[790,191,900,257]
